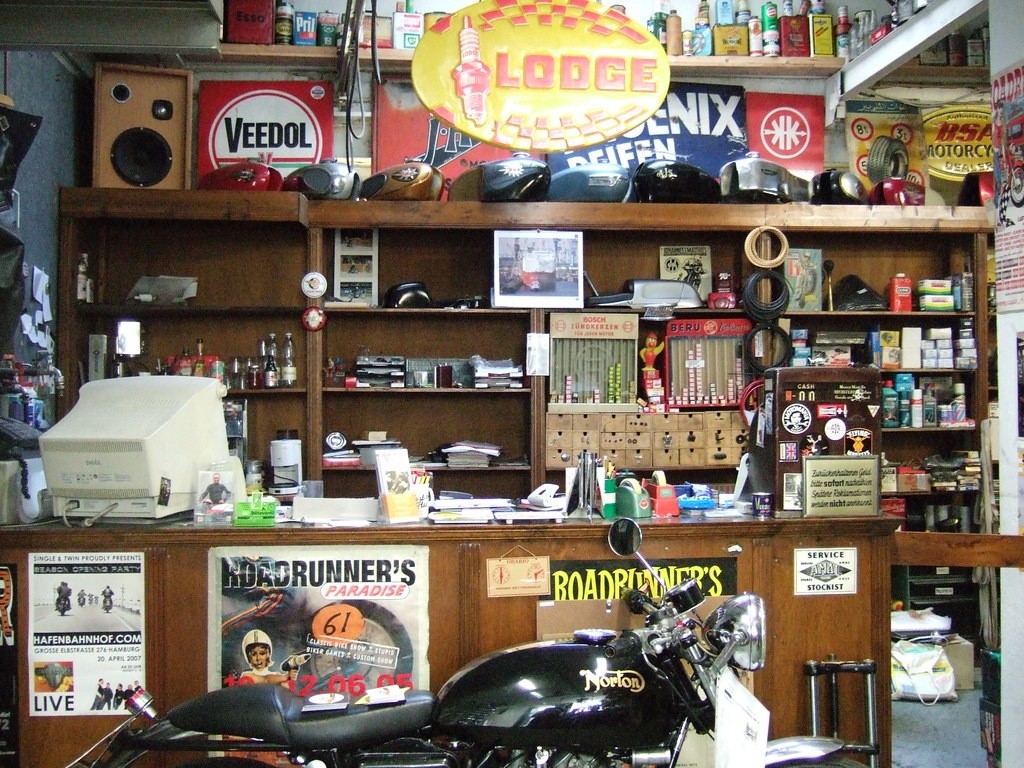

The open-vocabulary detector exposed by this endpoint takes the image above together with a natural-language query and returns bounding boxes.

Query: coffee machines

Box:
[268,438,303,495]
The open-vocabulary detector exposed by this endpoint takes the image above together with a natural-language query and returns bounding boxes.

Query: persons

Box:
[198,473,232,505]
[55,582,114,616]
[91,678,141,711]
[239,629,298,693]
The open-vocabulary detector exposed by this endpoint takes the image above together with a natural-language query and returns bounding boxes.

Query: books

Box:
[428,509,494,523]
[440,440,503,467]
[412,461,447,467]
[491,462,529,466]
[474,365,524,389]
[429,497,516,508]
[323,450,364,468]
[352,430,402,445]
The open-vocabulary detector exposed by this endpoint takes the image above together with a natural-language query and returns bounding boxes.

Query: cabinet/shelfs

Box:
[56,187,994,667]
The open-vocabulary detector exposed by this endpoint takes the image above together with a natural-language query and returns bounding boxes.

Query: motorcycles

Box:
[92,518,868,768]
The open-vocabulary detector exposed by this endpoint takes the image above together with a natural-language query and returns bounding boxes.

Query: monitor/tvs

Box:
[39,376,231,524]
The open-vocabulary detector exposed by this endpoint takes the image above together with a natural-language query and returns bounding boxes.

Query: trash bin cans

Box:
[974,649,1001,767]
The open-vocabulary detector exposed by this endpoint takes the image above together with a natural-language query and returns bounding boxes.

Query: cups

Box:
[752,492,772,519]
[298,479,324,497]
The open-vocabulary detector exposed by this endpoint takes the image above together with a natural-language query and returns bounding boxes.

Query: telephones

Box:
[0,415,44,448]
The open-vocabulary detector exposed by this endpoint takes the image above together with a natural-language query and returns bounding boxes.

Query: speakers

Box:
[92,60,194,191]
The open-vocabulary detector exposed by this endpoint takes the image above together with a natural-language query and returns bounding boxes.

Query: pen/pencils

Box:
[608,460,617,479]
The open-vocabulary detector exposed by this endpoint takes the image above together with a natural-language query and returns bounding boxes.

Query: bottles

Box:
[73,254,93,305]
[194,339,209,377]
[226,334,280,389]
[280,332,296,388]
[882,381,898,429]
[179,347,193,376]
[665,10,684,55]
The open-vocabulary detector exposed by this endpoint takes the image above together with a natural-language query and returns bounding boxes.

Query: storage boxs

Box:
[809,13,836,58]
[979,646,1002,704]
[89,335,108,381]
[916,278,952,295]
[881,467,898,493]
[896,474,931,493]
[977,697,1001,759]
[918,294,955,312]
[782,248,823,311]
[941,634,975,690]
[788,318,978,370]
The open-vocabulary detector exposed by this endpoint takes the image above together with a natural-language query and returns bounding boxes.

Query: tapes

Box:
[619,478,643,494]
[652,470,666,486]
